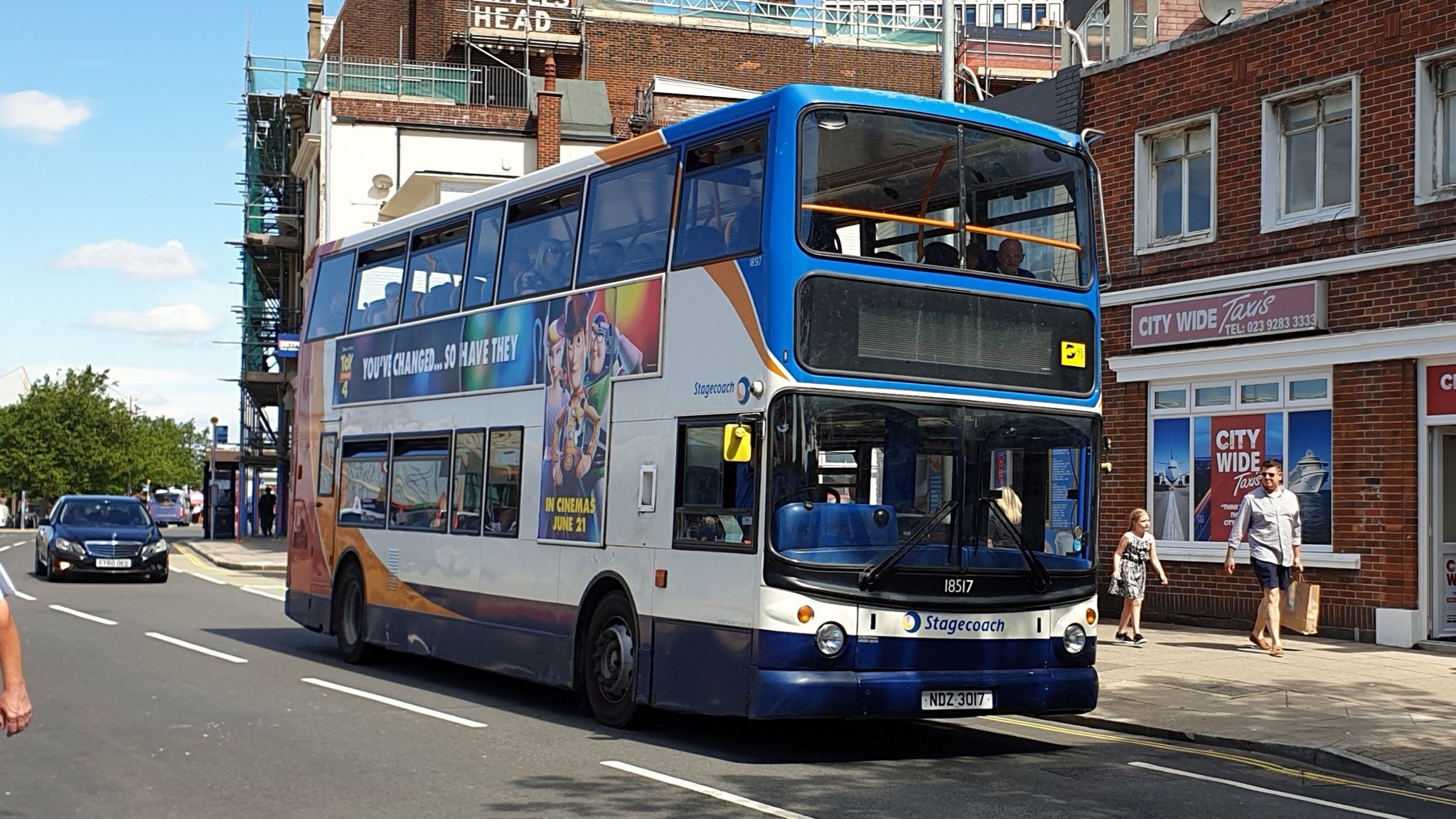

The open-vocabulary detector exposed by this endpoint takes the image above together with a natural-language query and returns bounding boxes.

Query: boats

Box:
[1288,449,1329,493]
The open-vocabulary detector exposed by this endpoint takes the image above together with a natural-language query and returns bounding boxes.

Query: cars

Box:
[34,495,170,584]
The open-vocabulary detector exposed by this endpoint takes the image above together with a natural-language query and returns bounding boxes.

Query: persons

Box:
[521,239,571,295]
[986,238,1038,279]
[987,486,1023,549]
[131,488,147,507]
[735,448,800,510]
[595,241,626,277]
[1112,507,1168,645]
[373,282,401,325]
[258,488,276,537]
[0,572,32,738]
[314,288,348,336]
[1224,459,1304,658]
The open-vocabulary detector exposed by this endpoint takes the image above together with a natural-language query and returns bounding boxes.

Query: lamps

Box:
[817,114,847,131]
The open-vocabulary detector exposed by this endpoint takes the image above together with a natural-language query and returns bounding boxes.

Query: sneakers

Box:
[1249,633,1271,650]
[1271,646,1284,657]
[1133,633,1147,644]
[1115,631,1133,643]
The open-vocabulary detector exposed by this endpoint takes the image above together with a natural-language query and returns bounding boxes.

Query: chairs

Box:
[364,214,1000,329]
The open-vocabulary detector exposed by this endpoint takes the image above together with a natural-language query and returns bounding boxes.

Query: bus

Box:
[283,84,1105,731]
[150,488,194,527]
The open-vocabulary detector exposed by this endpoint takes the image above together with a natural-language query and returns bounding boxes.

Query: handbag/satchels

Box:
[1279,572,1320,635]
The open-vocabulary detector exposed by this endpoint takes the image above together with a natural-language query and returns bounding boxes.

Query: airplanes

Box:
[1155,447,1188,487]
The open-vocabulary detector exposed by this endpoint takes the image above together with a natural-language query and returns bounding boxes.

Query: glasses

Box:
[1261,472,1278,477]
[547,249,565,254]
[385,292,400,299]
